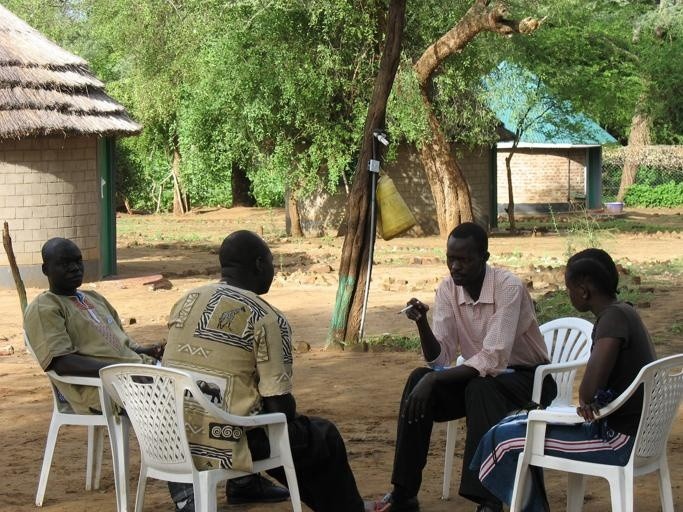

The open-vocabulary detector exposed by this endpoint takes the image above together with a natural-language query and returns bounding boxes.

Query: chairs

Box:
[441,317,594,499]
[509,351,683,512]
[22,329,127,506]
[98,361,303,512]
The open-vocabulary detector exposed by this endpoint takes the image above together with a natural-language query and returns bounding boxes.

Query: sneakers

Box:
[174,494,204,512]
[226,474,289,505]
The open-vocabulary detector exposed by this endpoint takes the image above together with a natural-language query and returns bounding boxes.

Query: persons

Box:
[24,237,290,512]
[469,249,658,512]
[382,223,556,512]
[161,230,391,512]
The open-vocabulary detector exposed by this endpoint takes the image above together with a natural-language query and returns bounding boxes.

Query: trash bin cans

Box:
[604,202,624,209]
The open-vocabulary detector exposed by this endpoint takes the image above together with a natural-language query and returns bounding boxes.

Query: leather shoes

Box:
[472,500,502,512]
[374,493,420,512]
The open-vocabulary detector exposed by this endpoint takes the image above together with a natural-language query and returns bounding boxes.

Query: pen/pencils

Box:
[396,299,424,314]
